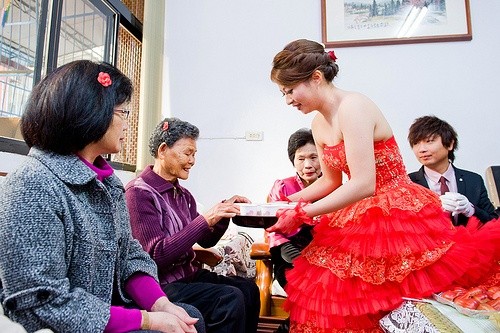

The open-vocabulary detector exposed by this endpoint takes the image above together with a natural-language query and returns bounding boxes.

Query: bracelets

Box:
[141,310,150,330]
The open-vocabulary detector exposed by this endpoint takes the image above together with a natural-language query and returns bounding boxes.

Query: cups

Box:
[234,201,304,217]
[445,192,460,208]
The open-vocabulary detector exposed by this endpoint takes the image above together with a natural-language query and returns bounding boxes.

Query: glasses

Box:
[113,110,130,121]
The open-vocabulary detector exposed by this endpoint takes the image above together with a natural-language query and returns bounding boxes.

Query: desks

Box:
[377,298,500,333]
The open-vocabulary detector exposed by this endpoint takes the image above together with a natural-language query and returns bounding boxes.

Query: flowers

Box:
[162,122,168,130]
[321,43,337,62]
[97,72,111,87]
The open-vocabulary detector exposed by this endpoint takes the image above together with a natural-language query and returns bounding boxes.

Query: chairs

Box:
[250,229,290,317]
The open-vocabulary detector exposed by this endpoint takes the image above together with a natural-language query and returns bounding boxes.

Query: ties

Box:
[438,176,458,226]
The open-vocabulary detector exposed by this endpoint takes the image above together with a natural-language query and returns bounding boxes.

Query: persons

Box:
[124,118,260,333]
[0,59,199,333]
[266,39,457,333]
[264,129,323,333]
[405,115,500,227]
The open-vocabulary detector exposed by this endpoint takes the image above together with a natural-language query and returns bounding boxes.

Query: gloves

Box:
[266,198,319,239]
[439,192,475,218]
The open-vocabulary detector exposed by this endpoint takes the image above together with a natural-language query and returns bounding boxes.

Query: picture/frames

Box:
[320,0,473,48]
